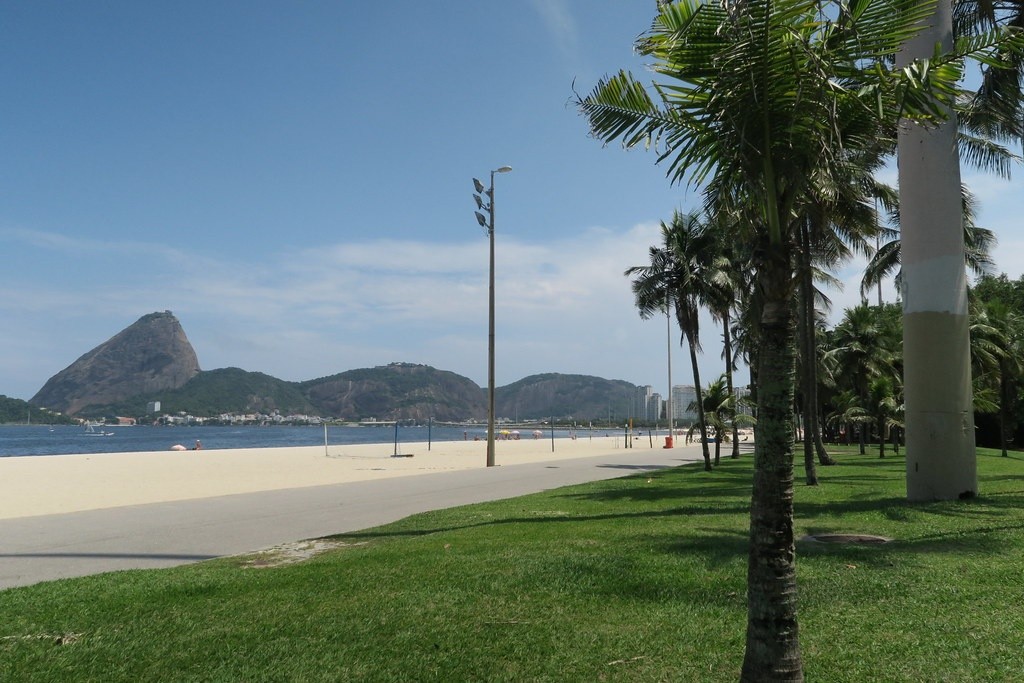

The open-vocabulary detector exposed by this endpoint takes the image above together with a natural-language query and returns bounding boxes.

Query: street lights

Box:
[472,166,512,466]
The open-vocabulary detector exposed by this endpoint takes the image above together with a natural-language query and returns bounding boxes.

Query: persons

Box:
[534,432,537,440]
[195,440,201,449]
[495,435,498,440]
[475,434,479,440]
[464,430,467,440]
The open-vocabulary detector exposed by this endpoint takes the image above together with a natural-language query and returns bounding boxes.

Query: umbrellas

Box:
[533,430,542,434]
[171,445,186,451]
[501,430,520,435]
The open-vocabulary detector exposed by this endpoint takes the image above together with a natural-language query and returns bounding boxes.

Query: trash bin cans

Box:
[665,437,673,449]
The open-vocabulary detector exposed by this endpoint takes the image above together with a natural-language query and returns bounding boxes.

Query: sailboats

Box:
[77,419,114,436]
[48,423,54,432]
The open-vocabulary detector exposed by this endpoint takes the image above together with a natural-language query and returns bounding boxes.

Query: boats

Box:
[700,438,716,442]
